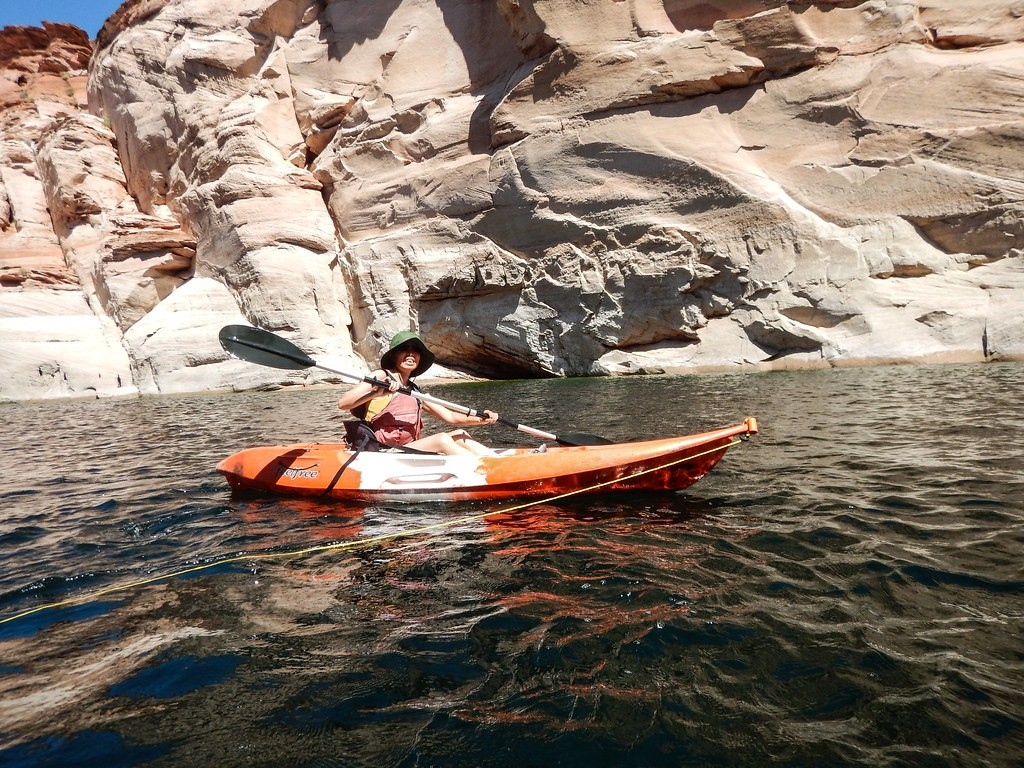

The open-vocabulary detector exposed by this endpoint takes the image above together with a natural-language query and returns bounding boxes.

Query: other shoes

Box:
[527,443,547,455]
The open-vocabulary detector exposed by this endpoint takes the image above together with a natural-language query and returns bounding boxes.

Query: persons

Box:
[337,329,547,456]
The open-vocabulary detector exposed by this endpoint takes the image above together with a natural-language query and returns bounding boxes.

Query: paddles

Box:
[217,324,616,448]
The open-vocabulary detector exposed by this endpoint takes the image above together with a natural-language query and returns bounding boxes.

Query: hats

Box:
[381,330,435,378]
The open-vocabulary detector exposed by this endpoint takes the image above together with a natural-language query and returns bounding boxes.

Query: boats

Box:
[216,418,758,501]
